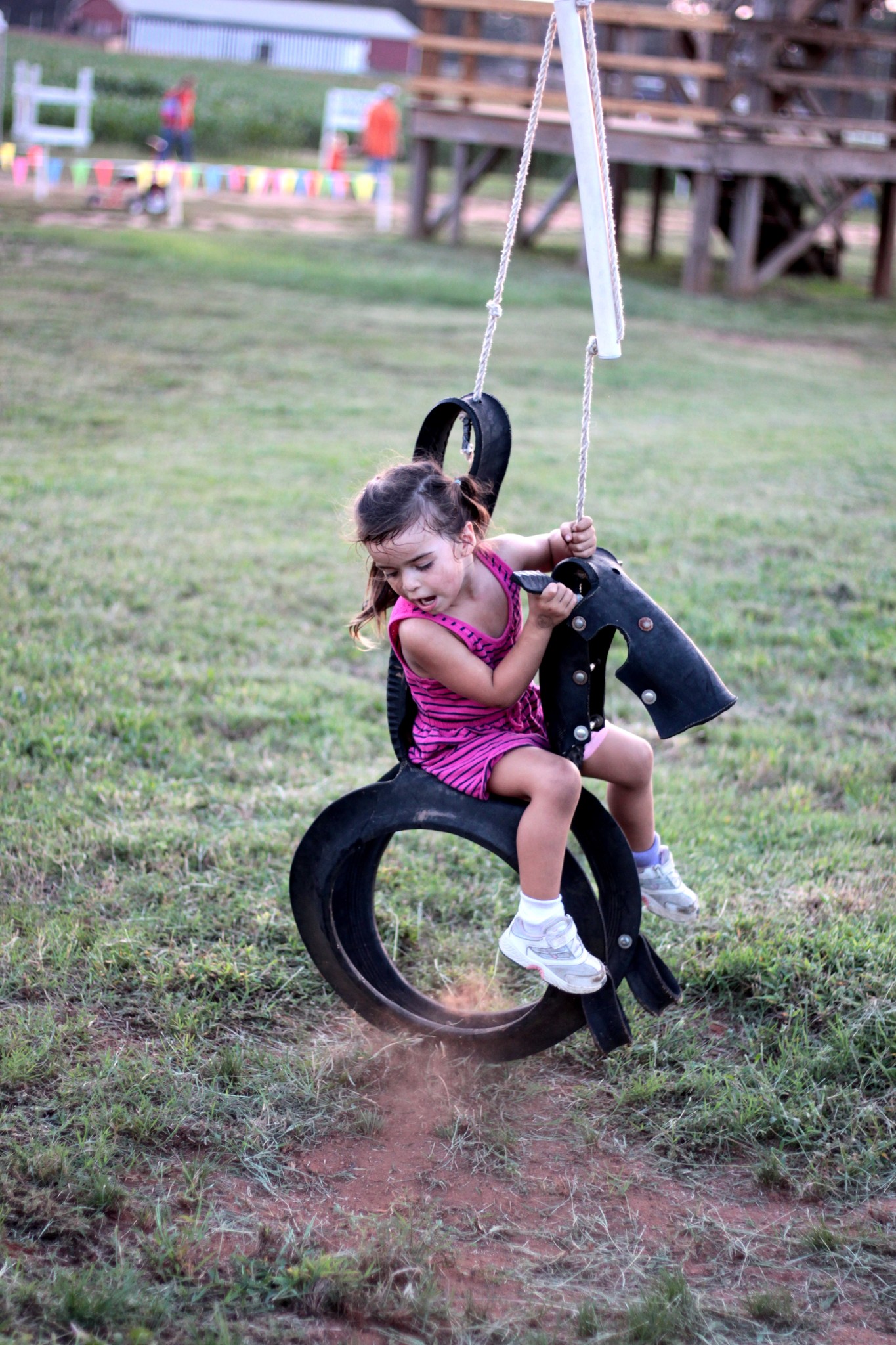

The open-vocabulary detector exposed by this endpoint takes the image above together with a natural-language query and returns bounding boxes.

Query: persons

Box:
[151,73,199,163]
[347,462,704,996]
[340,79,406,202]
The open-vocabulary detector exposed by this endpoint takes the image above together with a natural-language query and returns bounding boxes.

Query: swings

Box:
[289,0,734,1054]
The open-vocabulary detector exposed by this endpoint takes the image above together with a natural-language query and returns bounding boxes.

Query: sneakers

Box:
[499,913,607,994]
[637,844,699,923]
